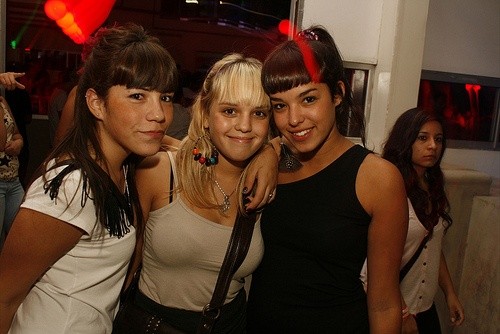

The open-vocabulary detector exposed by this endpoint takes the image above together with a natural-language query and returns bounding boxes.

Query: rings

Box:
[269,194,273,197]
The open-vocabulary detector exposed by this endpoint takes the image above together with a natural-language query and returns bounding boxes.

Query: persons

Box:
[0,22,280,334]
[361,107,465,334]
[245,24,410,334]
[0,62,78,252]
[112,52,271,334]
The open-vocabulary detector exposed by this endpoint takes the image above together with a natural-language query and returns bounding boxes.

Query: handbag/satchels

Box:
[113,293,219,334]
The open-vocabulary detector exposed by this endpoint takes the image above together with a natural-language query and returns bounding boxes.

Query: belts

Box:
[0,177,17,183]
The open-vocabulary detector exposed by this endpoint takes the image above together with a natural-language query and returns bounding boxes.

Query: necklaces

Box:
[208,170,236,212]
[122,166,131,206]
